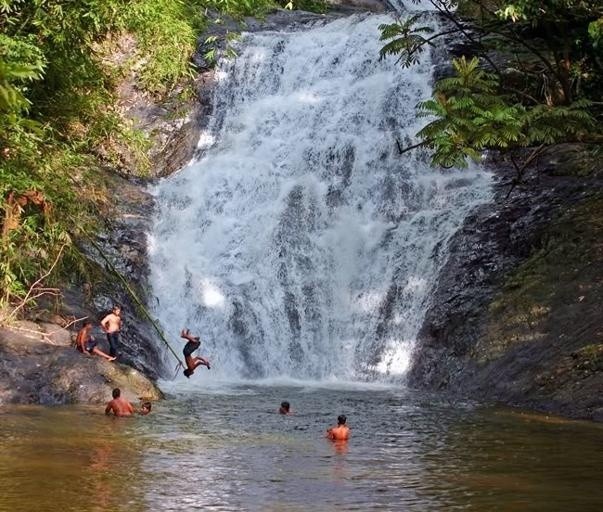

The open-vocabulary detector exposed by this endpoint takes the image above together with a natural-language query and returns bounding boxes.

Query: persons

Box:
[181,328,210,376]
[327,415,351,440]
[76,320,117,361]
[105,389,134,417]
[280,401,290,414]
[141,403,151,414]
[101,305,123,357]
